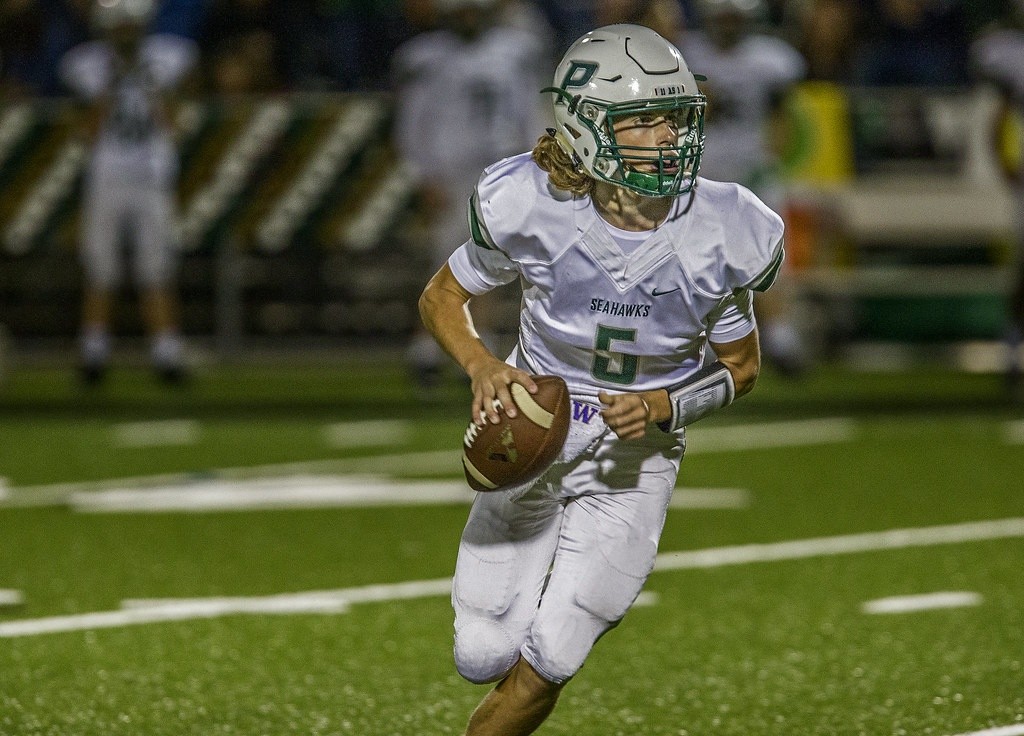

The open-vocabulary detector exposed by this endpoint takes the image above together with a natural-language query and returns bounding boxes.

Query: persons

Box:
[399,0,850,378]
[418,25,785,736]
[60,0,197,391]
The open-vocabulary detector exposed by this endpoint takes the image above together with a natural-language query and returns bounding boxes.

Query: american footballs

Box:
[463,374,571,491]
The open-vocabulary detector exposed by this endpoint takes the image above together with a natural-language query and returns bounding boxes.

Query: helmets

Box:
[543,26,705,198]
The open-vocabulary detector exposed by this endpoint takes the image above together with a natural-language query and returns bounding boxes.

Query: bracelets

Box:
[640,396,651,419]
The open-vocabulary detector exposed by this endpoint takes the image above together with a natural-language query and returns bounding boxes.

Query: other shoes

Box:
[162,363,190,388]
[81,365,101,389]
